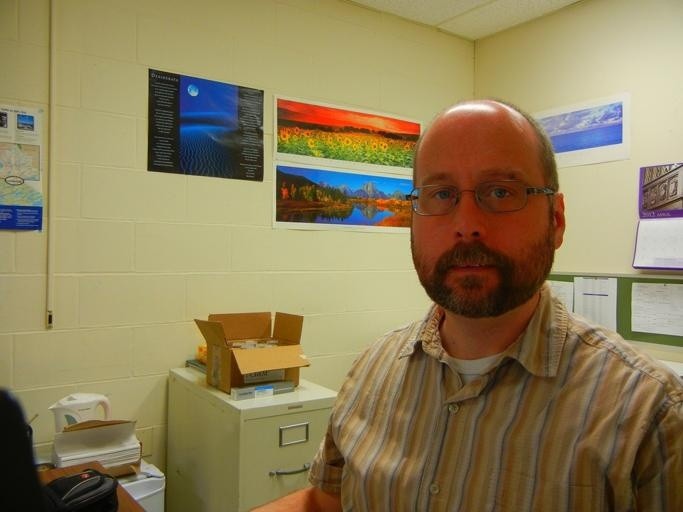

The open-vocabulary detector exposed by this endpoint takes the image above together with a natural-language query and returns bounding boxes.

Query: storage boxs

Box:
[196,311,311,395]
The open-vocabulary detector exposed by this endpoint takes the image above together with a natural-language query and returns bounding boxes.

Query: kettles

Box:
[48,392,111,432]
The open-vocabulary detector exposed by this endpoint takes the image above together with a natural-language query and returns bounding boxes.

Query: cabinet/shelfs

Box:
[166,367,342,512]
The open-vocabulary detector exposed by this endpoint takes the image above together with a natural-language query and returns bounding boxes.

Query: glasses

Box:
[401,180,556,215]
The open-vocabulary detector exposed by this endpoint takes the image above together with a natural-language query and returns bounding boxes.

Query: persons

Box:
[248,99,682,512]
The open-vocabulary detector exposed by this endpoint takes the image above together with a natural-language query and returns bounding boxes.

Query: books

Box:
[185,359,207,376]
